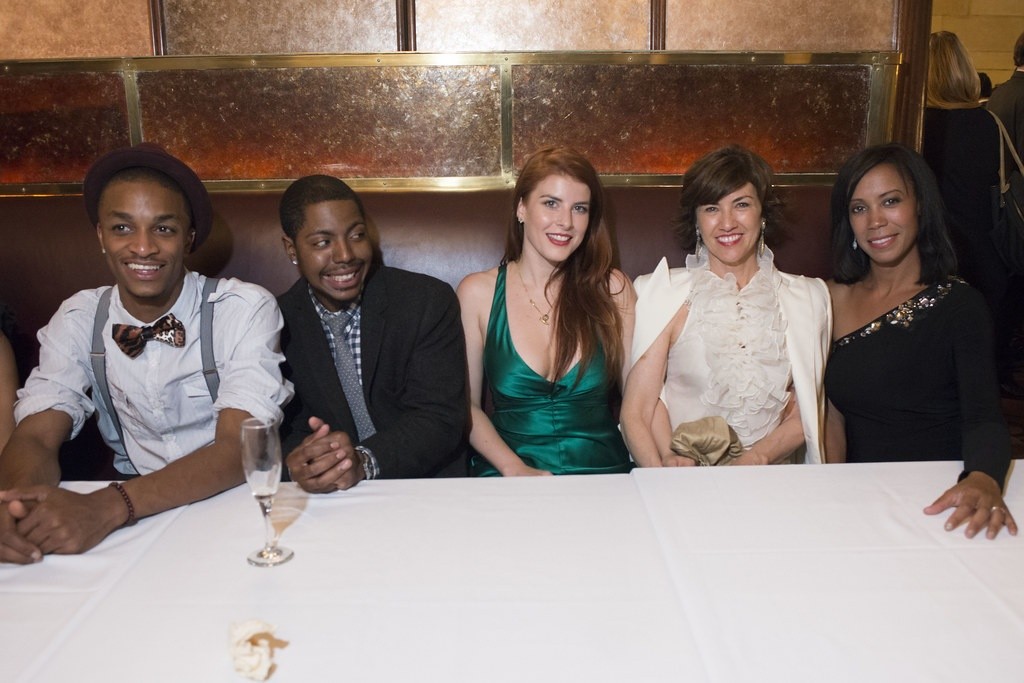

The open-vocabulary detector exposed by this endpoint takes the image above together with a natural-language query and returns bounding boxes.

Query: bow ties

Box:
[112,313,186,358]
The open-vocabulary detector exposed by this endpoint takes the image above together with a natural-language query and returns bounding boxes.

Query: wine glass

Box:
[241,417,292,567]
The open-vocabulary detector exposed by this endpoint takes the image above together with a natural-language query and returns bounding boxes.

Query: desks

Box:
[1,459,1024,683]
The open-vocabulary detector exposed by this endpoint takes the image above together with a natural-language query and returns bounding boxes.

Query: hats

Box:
[84,141,213,255]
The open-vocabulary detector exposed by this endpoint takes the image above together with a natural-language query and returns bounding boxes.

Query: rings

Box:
[991,506,1006,514]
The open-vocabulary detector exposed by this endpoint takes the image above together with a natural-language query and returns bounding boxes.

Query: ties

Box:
[323,312,378,442]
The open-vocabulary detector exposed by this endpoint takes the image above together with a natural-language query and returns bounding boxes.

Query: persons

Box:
[0,145,295,564]
[456,146,696,478]
[985,31,1024,287]
[619,145,834,467]
[276,174,474,494]
[976,72,993,104]
[924,30,1003,277]
[825,144,1018,540]
[0,329,17,451]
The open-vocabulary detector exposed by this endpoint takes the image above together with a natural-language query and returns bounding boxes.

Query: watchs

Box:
[359,451,374,481]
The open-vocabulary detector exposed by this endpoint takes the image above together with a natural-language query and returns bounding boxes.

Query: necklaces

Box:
[109,482,134,525]
[516,260,559,325]
